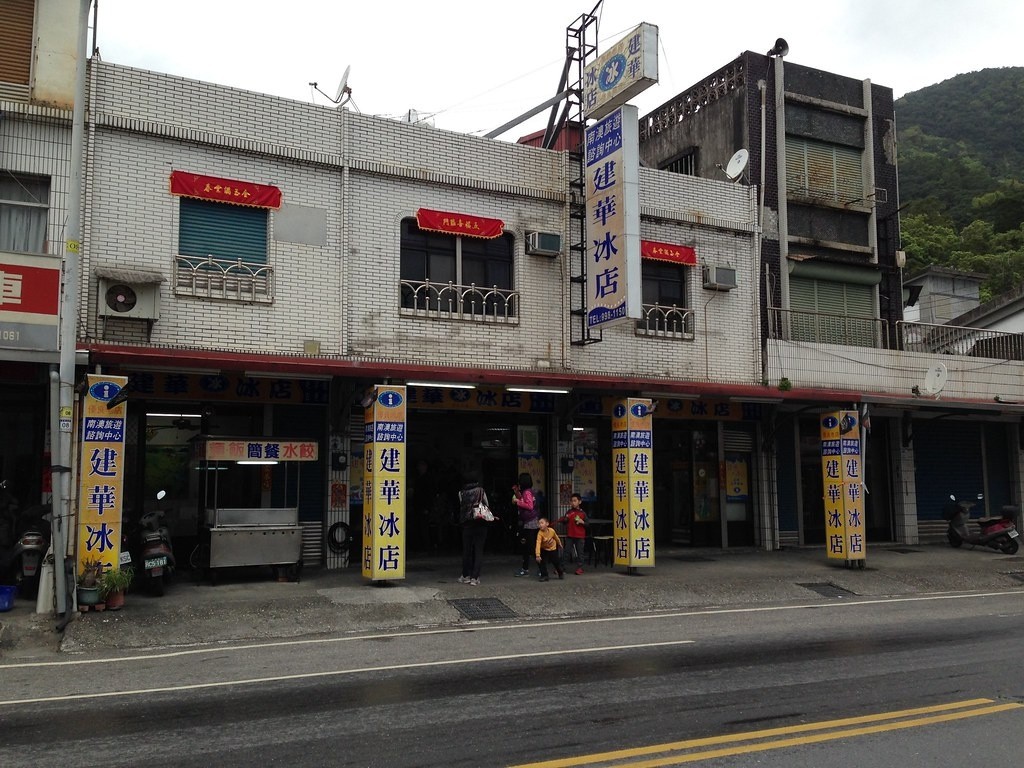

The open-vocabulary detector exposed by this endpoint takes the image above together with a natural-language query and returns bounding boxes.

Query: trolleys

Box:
[188,436,321,580]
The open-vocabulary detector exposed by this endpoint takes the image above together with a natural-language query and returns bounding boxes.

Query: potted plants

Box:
[77,556,104,604]
[99,567,133,611]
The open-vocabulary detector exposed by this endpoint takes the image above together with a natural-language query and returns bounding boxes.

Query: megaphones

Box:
[767,38,789,56]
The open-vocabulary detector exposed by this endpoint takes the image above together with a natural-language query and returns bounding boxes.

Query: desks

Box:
[585,519,614,564]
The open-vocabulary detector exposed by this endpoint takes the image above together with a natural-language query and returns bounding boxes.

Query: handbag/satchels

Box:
[473,488,495,522]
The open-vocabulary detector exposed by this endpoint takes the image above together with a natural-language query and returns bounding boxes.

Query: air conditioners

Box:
[98,277,162,324]
[524,230,561,258]
[701,265,738,293]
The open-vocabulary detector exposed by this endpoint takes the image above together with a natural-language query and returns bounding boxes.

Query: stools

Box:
[588,536,615,568]
[557,533,578,559]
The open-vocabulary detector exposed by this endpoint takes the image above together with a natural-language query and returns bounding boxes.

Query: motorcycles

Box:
[118,515,131,575]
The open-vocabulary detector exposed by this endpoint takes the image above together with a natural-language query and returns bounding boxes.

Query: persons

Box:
[512,473,542,577]
[453,480,489,586]
[414,460,433,552]
[535,517,564,582]
[554,493,590,575]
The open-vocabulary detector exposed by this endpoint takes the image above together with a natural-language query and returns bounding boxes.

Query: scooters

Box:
[14,502,56,591]
[0,478,25,548]
[947,494,1019,554]
[136,490,176,595]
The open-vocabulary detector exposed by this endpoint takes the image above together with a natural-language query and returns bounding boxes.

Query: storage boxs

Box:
[0,585,17,611]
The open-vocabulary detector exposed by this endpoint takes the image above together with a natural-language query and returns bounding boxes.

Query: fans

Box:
[145,417,220,432]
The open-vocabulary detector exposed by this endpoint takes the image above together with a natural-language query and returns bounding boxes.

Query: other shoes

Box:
[574,568,584,574]
[559,575,564,579]
[538,576,549,582]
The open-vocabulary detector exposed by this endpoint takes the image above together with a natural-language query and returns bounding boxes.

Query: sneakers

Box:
[514,567,530,577]
[456,575,471,584]
[470,577,480,586]
